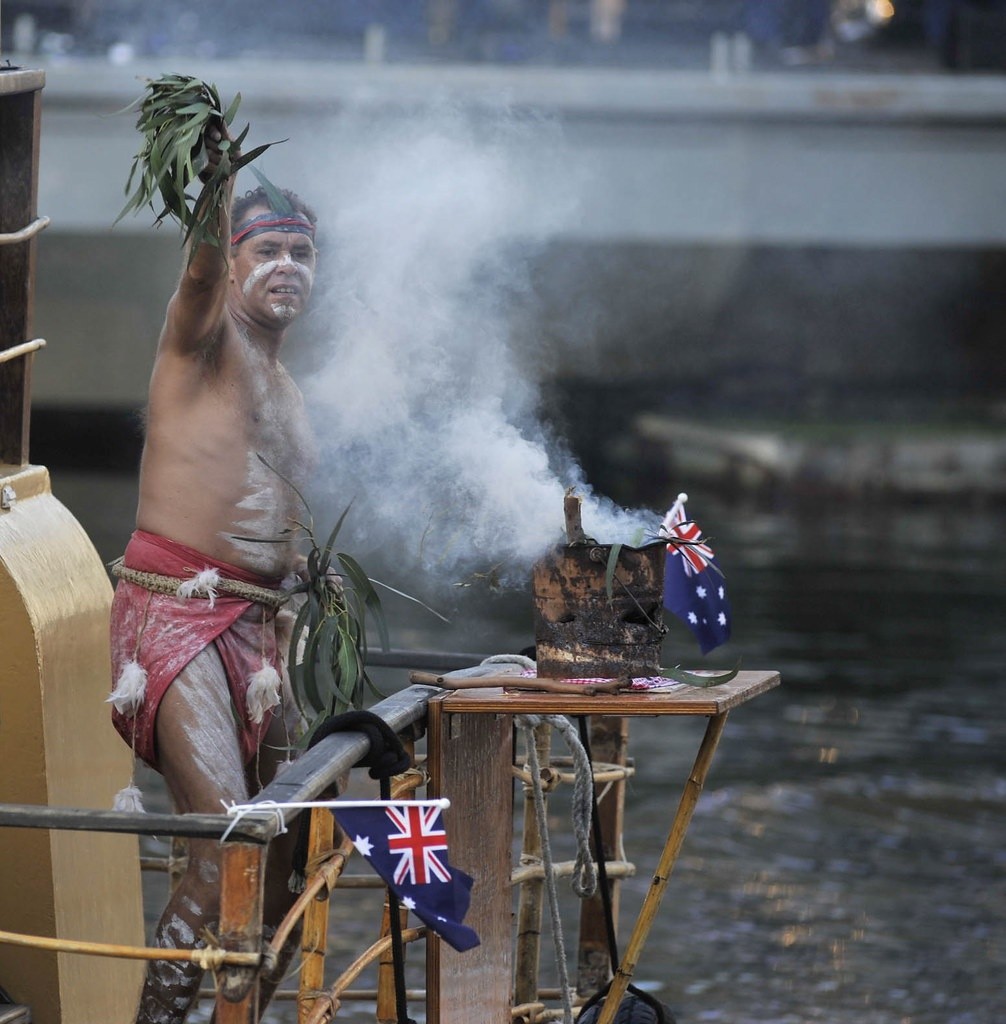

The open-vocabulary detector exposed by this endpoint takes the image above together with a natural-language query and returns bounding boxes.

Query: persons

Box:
[108,104,346,1021]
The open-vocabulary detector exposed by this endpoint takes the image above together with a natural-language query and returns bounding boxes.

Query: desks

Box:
[441,668,784,1024]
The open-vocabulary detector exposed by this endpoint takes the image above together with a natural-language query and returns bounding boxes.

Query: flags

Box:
[329,807,482,953]
[661,503,737,648]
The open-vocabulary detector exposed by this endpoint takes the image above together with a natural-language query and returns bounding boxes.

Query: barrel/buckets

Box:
[533,542,669,680]
